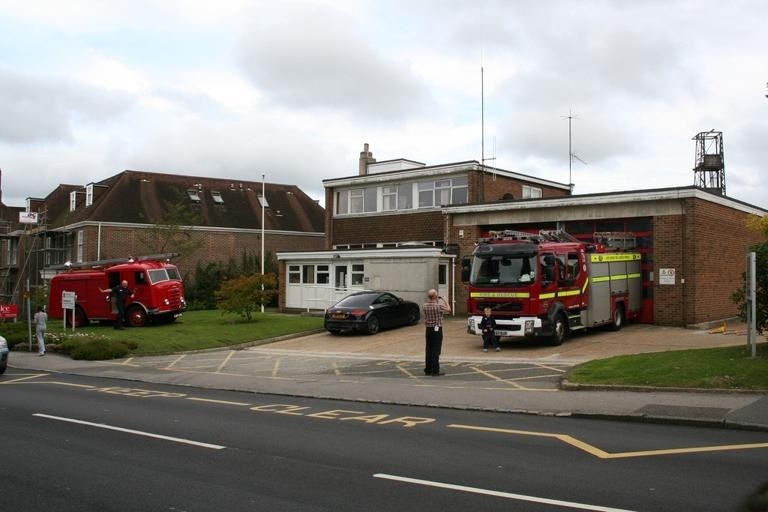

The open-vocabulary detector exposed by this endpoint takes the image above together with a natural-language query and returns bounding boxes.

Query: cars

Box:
[320,290,422,337]
[0,334,10,376]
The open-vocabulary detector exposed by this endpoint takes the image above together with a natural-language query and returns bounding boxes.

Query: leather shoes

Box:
[425,371,446,376]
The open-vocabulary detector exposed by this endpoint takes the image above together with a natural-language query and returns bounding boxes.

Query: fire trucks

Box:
[459,226,646,351]
[42,248,189,330]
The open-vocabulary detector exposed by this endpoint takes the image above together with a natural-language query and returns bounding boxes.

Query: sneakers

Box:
[495,347,501,351]
[37,350,46,356]
[483,348,488,352]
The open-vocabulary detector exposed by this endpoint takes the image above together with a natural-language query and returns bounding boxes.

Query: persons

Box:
[31,306,49,356]
[480,307,502,352]
[98,280,135,330]
[421,289,451,376]
